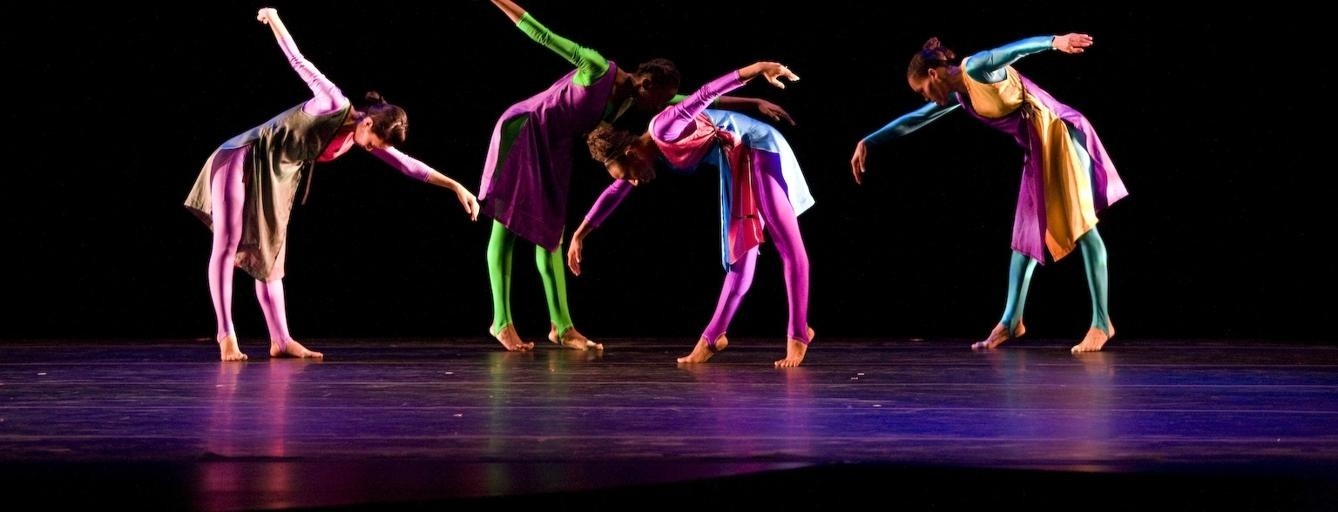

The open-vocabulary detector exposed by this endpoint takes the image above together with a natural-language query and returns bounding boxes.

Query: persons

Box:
[568,60,817,367]
[180,7,483,361]
[849,30,1131,353]
[477,0,800,352]
